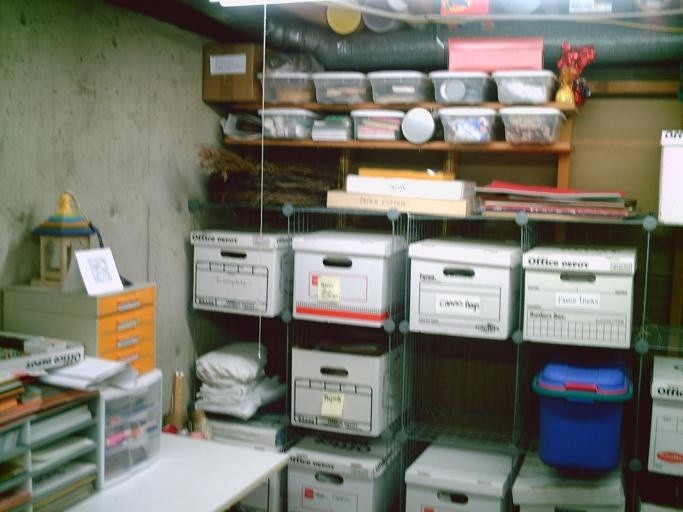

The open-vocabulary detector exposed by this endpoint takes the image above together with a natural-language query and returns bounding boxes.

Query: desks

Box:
[64,432,290,512]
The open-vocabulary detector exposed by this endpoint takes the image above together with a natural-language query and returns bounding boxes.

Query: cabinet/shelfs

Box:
[187,37,598,212]
[190,198,410,510]
[393,204,656,511]
[628,209,683,512]
[0,389,99,512]
[0,283,157,378]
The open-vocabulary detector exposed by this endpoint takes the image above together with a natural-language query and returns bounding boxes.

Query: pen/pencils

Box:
[107,399,158,450]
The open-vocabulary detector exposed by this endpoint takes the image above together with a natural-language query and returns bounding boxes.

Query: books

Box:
[325,165,477,218]
[1,381,99,512]
[472,180,638,222]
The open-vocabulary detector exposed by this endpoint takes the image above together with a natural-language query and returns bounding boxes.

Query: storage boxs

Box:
[636,488,683,511]
[289,334,414,438]
[72,367,163,492]
[643,354,683,478]
[284,430,411,510]
[189,227,302,321]
[527,346,636,473]
[406,234,521,341]
[522,243,639,351]
[509,437,627,511]
[290,228,424,329]
[191,409,305,511]
[401,428,529,511]
